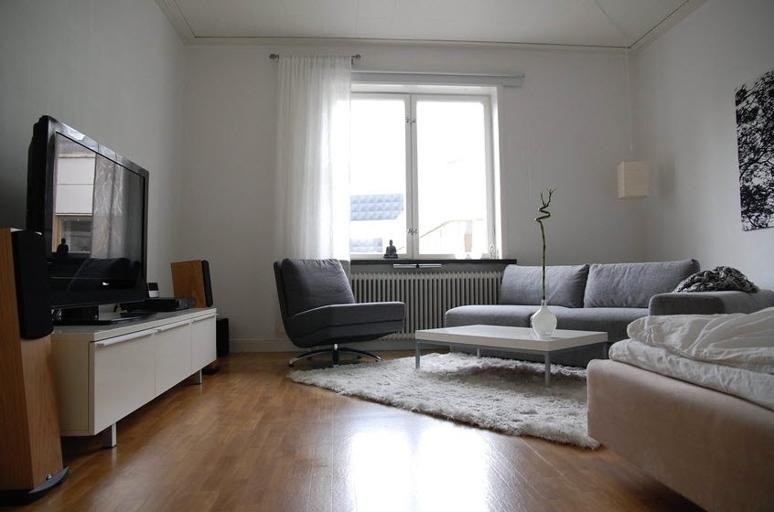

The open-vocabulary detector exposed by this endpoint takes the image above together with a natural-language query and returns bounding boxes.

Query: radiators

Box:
[350,271,503,341]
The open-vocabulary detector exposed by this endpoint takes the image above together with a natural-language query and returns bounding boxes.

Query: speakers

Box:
[1,226,70,496]
[169,259,214,309]
[216,316,229,357]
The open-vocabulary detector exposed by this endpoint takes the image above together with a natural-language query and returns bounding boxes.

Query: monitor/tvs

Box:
[25,113,150,328]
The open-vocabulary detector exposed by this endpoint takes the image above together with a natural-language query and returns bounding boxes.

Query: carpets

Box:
[287,352,601,453]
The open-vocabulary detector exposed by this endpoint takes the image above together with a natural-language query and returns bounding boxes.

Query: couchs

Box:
[443,258,774,368]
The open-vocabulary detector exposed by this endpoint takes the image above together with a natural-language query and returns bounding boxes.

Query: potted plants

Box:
[529,185,559,337]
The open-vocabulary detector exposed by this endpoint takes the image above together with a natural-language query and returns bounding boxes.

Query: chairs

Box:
[273,259,406,371]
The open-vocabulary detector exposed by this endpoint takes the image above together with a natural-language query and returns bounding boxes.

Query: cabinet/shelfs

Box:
[52,306,218,449]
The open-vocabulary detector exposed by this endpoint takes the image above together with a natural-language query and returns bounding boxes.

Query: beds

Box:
[588,306,773,510]
